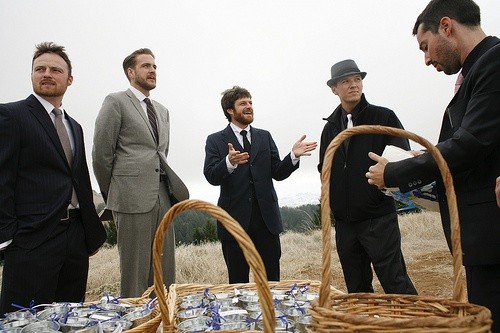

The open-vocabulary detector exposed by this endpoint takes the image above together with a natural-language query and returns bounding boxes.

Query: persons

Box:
[204,87,317,284]
[317,59,411,295]
[0,42,107,317]
[365,0,500,333]
[92,48,190,299]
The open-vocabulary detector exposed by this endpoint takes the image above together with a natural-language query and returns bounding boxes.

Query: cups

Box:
[176,286,318,333]
[0,295,153,333]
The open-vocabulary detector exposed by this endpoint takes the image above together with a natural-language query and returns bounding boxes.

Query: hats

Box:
[326,59,367,88]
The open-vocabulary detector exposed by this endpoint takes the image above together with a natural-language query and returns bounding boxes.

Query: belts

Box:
[60,209,82,221]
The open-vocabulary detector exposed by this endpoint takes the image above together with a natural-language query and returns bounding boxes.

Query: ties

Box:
[345,114,353,130]
[240,130,255,166]
[454,73,465,95]
[142,98,161,147]
[50,109,79,208]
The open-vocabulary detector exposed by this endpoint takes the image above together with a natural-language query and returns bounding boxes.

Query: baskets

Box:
[84,283,168,332]
[306,125,494,333]
[152,200,379,333]
